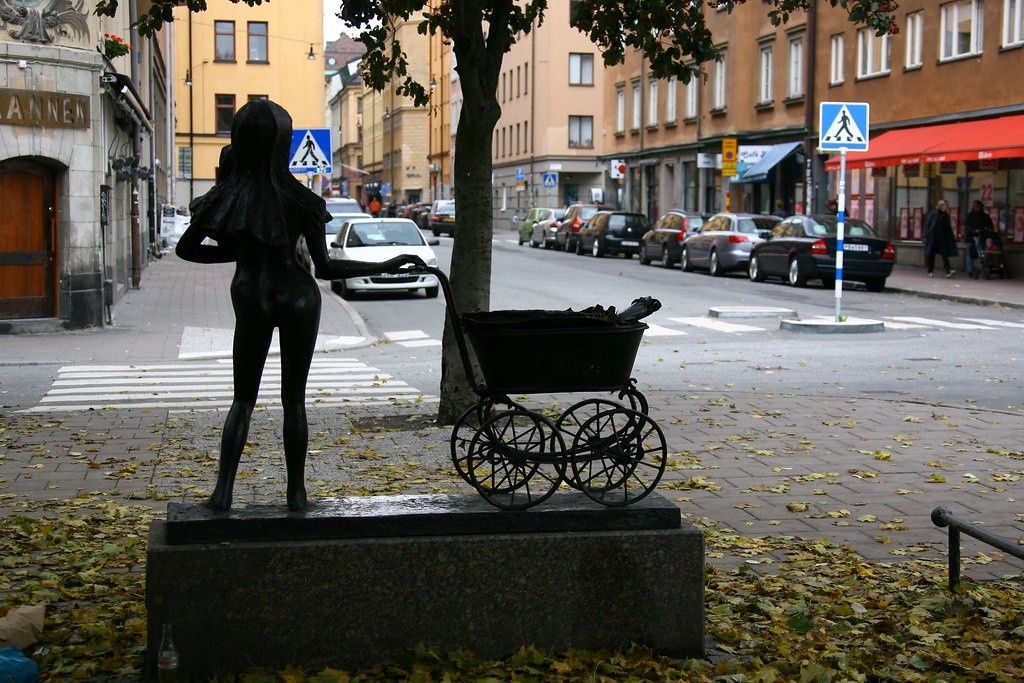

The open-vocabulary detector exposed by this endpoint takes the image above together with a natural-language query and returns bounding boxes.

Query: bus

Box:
[329,217,441,300]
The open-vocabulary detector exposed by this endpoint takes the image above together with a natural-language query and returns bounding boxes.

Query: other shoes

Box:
[928,272,935,277]
[969,271,978,279]
[946,270,956,277]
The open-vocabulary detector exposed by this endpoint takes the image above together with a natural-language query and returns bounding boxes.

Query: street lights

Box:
[428,163,441,201]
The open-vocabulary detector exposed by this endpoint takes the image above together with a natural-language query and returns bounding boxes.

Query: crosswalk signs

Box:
[541,172,559,189]
[288,129,333,173]
[819,102,870,152]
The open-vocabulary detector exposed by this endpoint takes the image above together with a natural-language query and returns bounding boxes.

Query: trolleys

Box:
[407,263,667,513]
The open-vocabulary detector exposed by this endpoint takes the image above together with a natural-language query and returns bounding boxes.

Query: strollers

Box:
[971,228,1012,280]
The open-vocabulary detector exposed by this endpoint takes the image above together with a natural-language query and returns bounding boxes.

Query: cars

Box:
[431,199,457,238]
[681,212,784,277]
[530,209,566,250]
[381,199,450,229]
[518,208,549,246]
[576,210,651,257]
[748,214,898,293]
[324,211,384,256]
[638,209,715,270]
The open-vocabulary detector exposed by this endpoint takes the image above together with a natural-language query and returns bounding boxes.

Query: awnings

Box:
[822,114,1024,172]
[729,142,802,183]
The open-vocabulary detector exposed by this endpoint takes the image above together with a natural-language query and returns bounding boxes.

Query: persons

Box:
[825,199,849,217]
[387,198,397,217]
[921,199,959,278]
[359,198,365,213]
[985,238,996,252]
[963,200,993,279]
[176,99,428,511]
[370,197,381,218]
[771,199,787,217]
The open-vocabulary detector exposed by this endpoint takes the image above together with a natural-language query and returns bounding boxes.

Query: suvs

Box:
[555,204,616,253]
[321,197,364,213]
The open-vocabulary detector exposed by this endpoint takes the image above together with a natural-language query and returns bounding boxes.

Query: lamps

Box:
[384,105,391,119]
[304,42,318,61]
[429,74,438,89]
[338,116,362,134]
[180,70,194,87]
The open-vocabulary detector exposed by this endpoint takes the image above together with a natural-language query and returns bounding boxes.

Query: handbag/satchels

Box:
[969,244,978,258]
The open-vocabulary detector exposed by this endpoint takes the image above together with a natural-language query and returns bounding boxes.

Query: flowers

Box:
[105,32,134,60]
